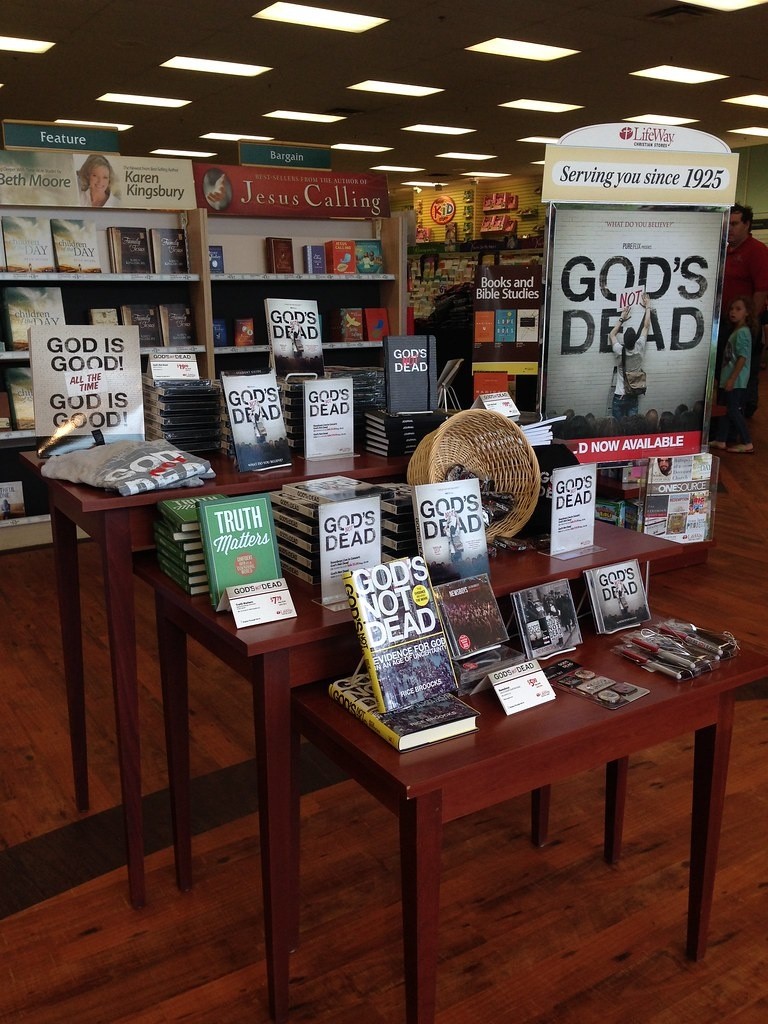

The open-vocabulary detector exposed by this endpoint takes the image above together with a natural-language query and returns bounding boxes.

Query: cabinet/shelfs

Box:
[409,247,544,320]
[596,469,707,579]
[0,208,408,447]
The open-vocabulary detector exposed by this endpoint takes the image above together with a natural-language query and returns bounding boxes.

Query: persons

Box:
[608,293,651,418]
[77,153,119,207]
[656,457,672,482]
[706,201,767,454]
[545,401,705,440]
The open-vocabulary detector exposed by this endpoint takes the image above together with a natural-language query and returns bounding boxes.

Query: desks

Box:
[130,519,682,1024]
[18,449,413,909]
[285,610,768,1024]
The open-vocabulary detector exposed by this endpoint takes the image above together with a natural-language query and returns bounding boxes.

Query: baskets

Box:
[406,408,541,545]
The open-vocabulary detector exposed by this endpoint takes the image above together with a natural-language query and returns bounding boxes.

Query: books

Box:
[506,409,566,448]
[152,476,492,605]
[0,207,446,467]
[328,557,480,753]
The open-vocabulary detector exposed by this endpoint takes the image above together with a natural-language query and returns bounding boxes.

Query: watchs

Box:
[618,317,623,323]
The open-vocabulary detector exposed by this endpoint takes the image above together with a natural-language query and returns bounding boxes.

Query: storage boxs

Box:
[325,241,356,274]
[331,307,362,342]
[595,498,644,532]
[599,461,648,483]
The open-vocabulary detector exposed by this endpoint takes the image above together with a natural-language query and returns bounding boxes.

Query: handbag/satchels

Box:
[624,370,647,396]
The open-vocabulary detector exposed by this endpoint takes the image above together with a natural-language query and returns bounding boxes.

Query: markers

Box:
[613,617,741,681]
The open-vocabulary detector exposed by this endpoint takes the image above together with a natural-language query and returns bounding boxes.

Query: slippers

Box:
[708,440,726,450]
[726,444,755,453]
